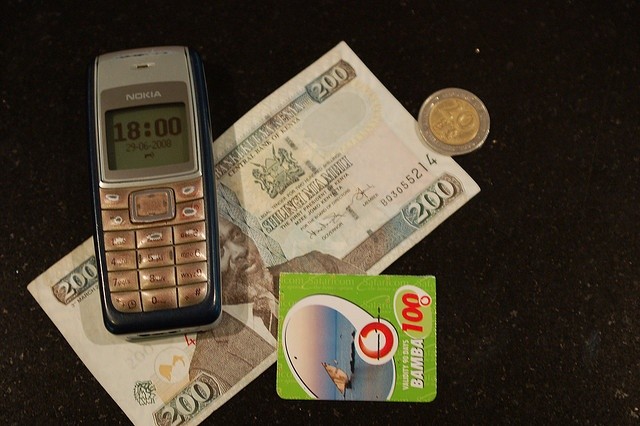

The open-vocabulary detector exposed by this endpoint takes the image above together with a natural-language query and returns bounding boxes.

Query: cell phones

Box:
[83,45,223,341]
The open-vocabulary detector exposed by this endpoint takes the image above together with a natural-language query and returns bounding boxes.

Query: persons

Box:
[187,214,378,398]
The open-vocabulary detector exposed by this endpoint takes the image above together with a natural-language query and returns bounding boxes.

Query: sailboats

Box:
[319,361,352,398]
